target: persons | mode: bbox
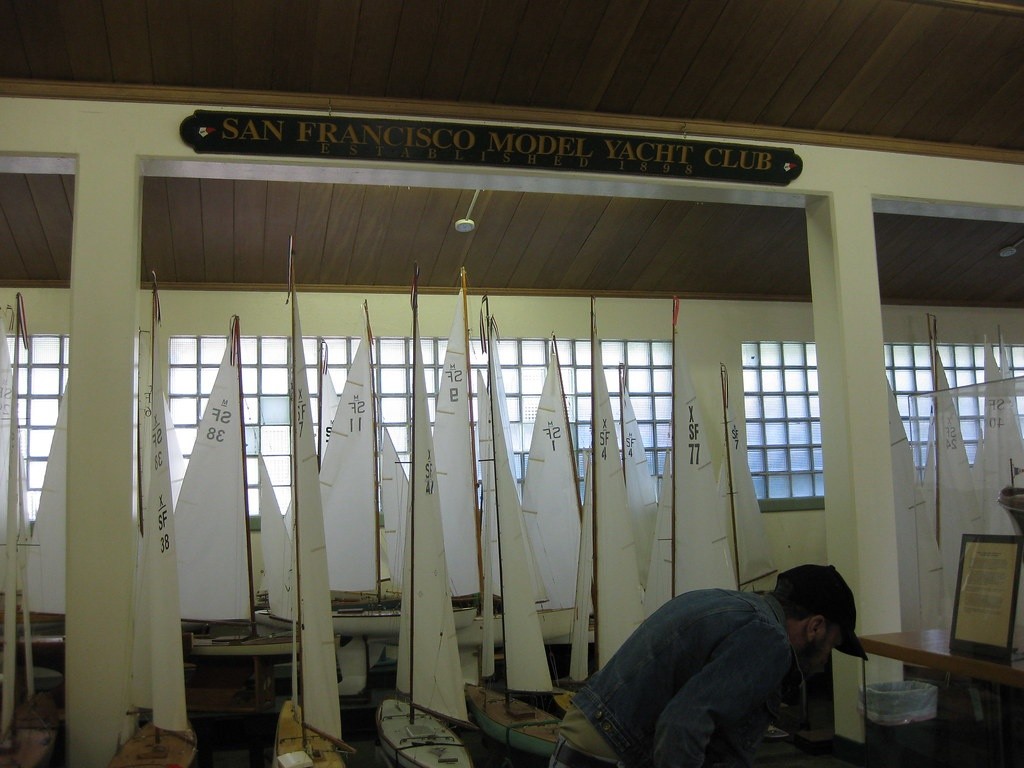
[547,565,869,767]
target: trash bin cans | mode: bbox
[859,680,938,768]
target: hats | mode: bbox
[774,565,868,661]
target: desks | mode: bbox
[862,627,1024,768]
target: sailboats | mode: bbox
[0,236,1024,768]
